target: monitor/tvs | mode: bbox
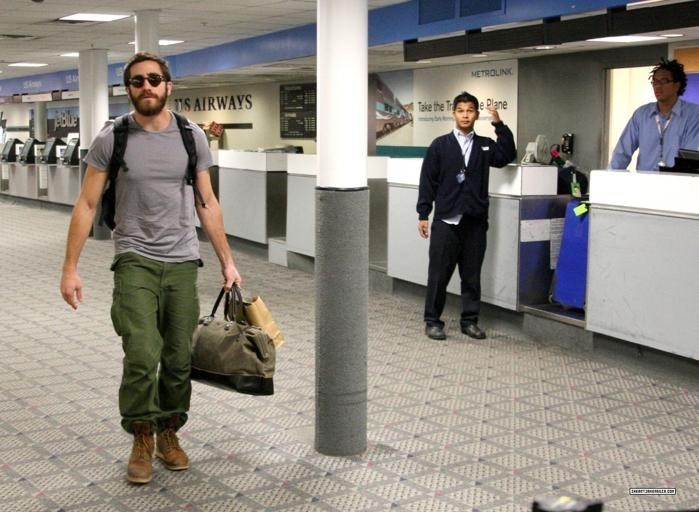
[20,138,38,161]
[40,137,57,162]
[2,138,25,161]
[64,138,79,162]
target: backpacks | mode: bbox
[101,111,199,231]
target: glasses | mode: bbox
[650,77,672,86]
[129,72,166,88]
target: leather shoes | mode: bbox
[461,323,486,339]
[425,324,446,340]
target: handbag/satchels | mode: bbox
[192,284,276,396]
[228,284,285,350]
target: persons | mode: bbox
[414,90,517,340]
[59,47,245,484]
[604,57,698,174]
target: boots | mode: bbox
[127,422,155,483]
[155,428,189,470]
